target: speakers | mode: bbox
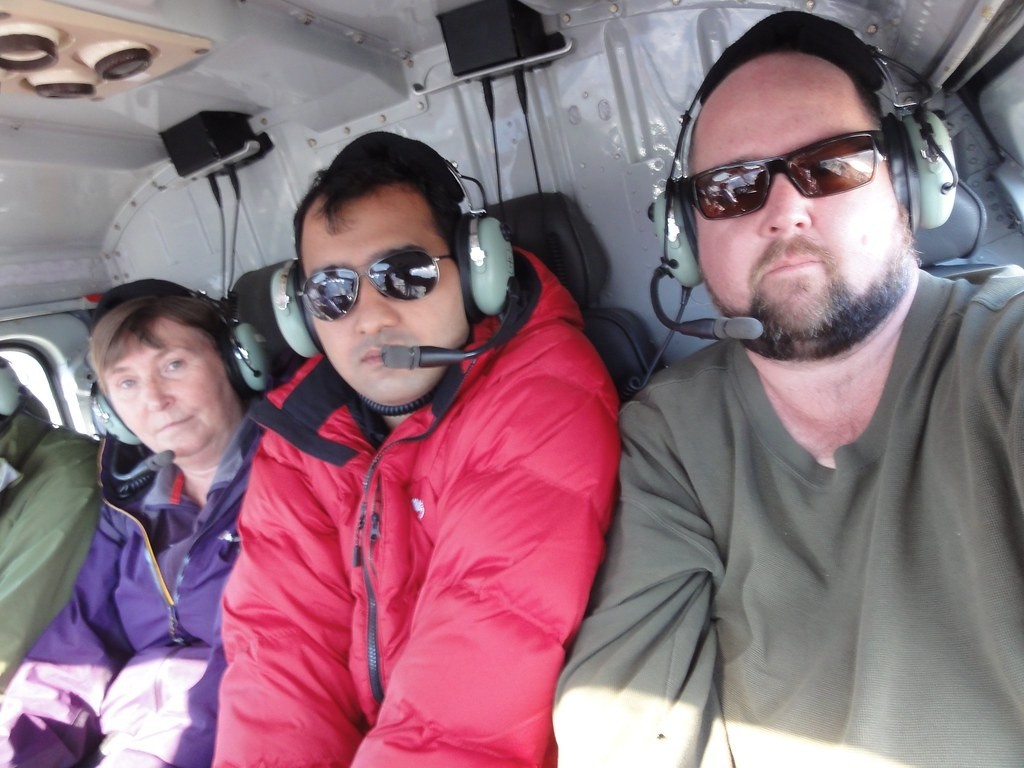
[437,0,547,77]
[158,110,257,176]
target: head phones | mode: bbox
[648,10,959,287]
[270,147,515,357]
[0,357,22,421]
[88,278,271,445]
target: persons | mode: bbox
[0,356,102,705]
[212,130,620,767]
[552,9,1023,766]
[1,278,266,768]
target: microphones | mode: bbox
[382,277,520,369]
[649,265,763,341]
[110,441,176,482]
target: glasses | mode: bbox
[298,251,455,321]
[681,129,889,220]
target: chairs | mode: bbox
[219,262,305,394]
[914,175,1007,286]
[473,189,658,414]
